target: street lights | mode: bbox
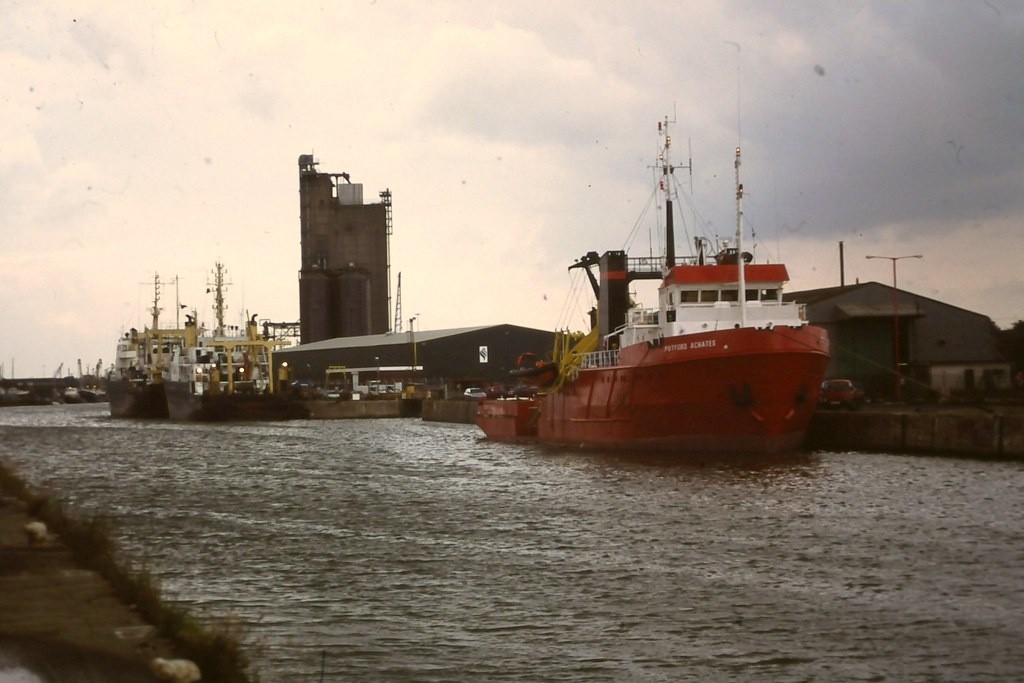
[865,254,923,399]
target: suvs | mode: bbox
[507,384,537,397]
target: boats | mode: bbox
[64,263,291,422]
[475,103,831,452]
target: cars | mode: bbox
[464,388,487,398]
[817,379,864,408]
[484,386,513,398]
